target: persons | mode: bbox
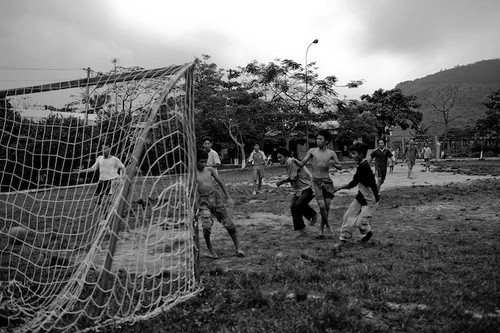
[74,144,126,224]
[368,140,395,197]
[420,142,432,173]
[292,130,342,238]
[202,138,221,175]
[247,143,270,196]
[402,138,422,179]
[388,147,396,172]
[189,152,245,257]
[334,142,379,248]
[275,148,318,235]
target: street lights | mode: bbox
[304,39,319,154]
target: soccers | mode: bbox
[9,227,27,245]
